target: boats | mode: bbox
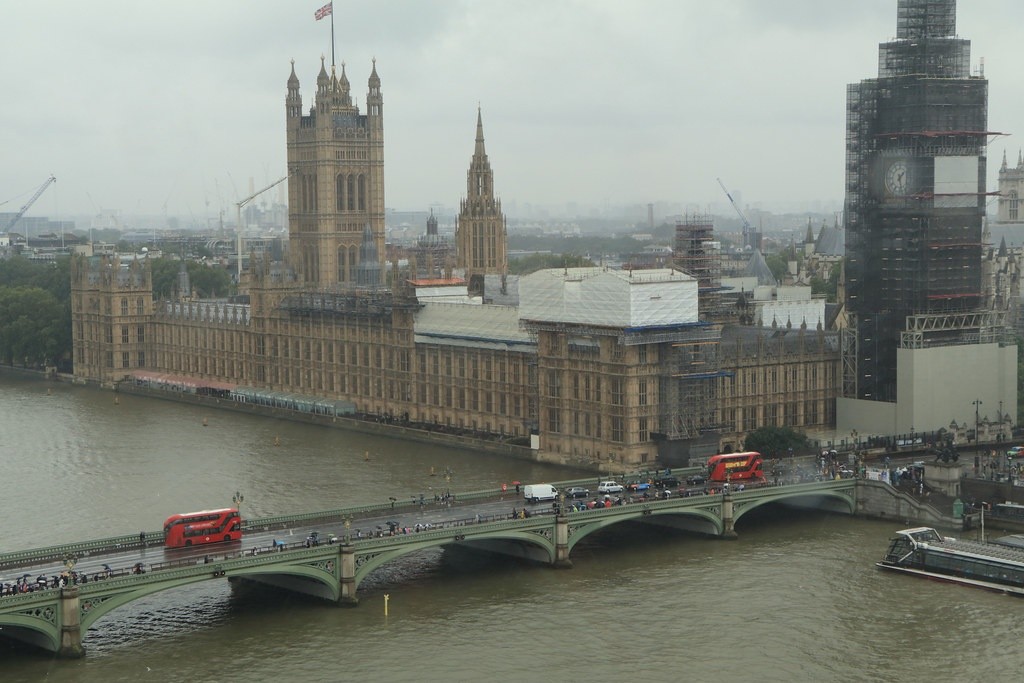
[875,526,1023,598]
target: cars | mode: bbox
[1006,446,1024,458]
[686,474,708,485]
[564,486,590,499]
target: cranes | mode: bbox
[0,175,58,238]
[236,172,295,287]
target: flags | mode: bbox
[313,2,334,21]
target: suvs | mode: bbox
[597,480,624,494]
[654,475,682,488]
[626,480,651,492]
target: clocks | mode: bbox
[885,160,915,196]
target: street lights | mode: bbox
[999,400,1003,456]
[724,467,734,496]
[62,550,79,588]
[971,397,982,454]
[232,490,245,511]
[556,486,567,517]
[910,425,914,463]
[341,513,354,545]
[981,501,988,542]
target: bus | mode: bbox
[162,508,244,548]
[706,451,764,481]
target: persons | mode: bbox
[0,529,154,598]
[202,466,745,564]
[758,450,936,506]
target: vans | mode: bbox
[524,483,559,502]
